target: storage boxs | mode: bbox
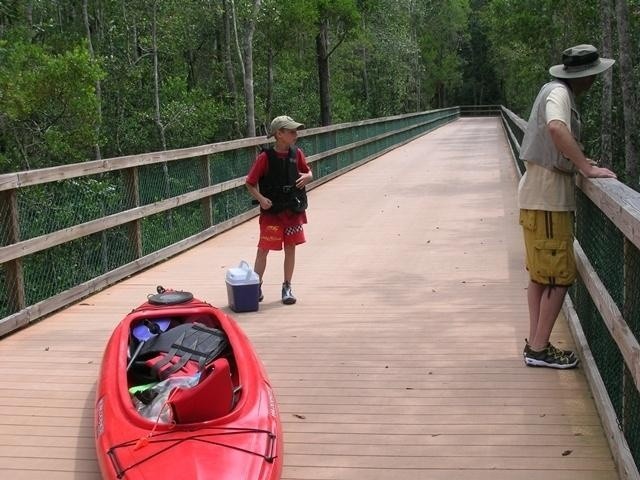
[223,259,263,312]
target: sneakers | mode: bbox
[522,337,579,369]
[281,282,296,304]
[257,281,264,301]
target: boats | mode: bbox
[92,286,284,480]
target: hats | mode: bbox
[266,116,305,139]
[548,44,616,79]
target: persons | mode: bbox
[244,115,314,305]
[517,43,619,370]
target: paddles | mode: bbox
[126,316,172,372]
